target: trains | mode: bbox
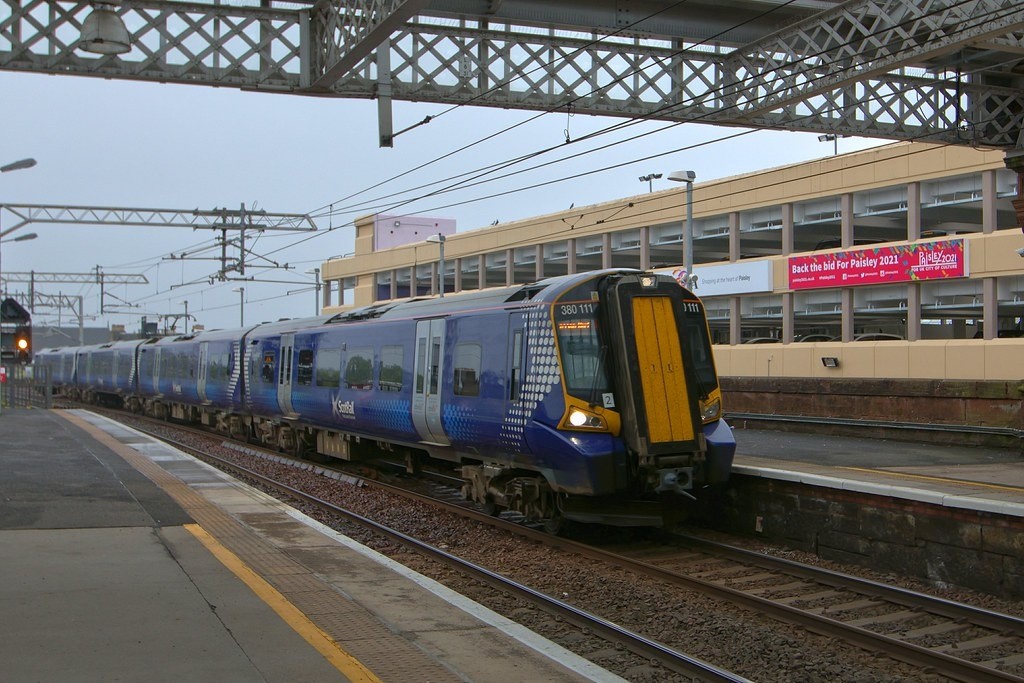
[34,268,735,535]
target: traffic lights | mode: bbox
[16,325,31,350]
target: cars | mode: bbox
[739,332,903,343]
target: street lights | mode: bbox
[426,233,446,298]
[667,170,695,295]
[0,233,38,246]
[233,288,244,326]
